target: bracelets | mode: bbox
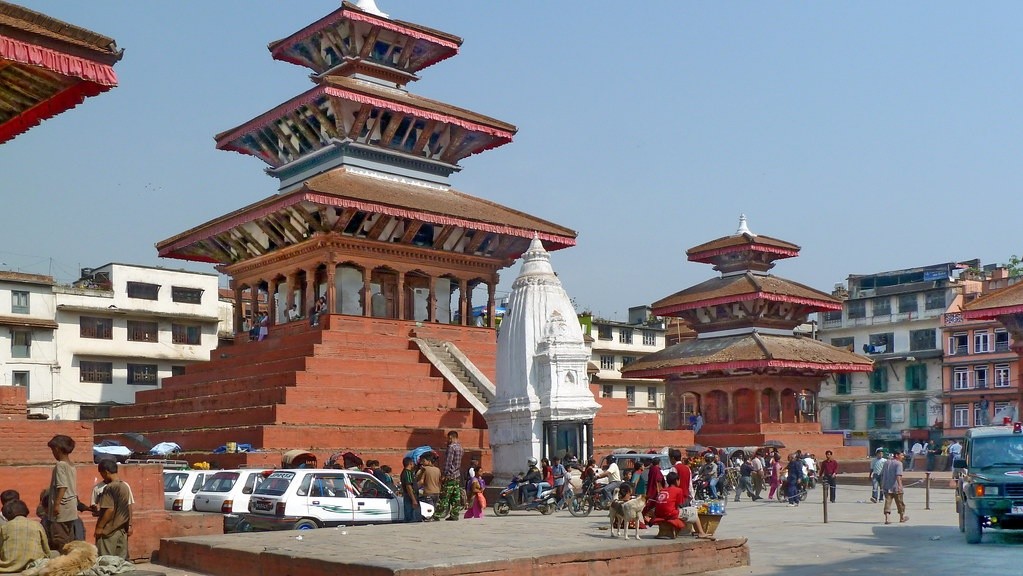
[129,525,132,526]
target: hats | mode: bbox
[774,452,781,459]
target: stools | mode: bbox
[654,522,681,540]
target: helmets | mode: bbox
[606,454,617,464]
[704,453,715,464]
[528,456,537,467]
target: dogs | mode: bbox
[607,494,650,540]
[20,540,98,576]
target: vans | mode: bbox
[598,452,675,490]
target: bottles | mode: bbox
[710,501,725,515]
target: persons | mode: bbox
[434,431,464,521]
[400,457,421,522]
[870,446,904,502]
[908,438,963,470]
[518,408,818,529]
[246,296,328,343]
[475,313,486,327]
[327,460,424,505]
[655,471,712,538]
[880,448,910,523]
[47,435,78,556]
[0,489,20,524]
[90,476,135,537]
[40,489,96,542]
[93,458,130,560]
[416,453,441,509]
[819,450,839,503]
[464,466,487,518]
[0,499,51,573]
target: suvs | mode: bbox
[955,417,1022,544]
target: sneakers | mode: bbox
[879,499,883,503]
[870,497,877,503]
[786,504,799,507]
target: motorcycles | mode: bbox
[692,466,727,502]
[494,472,558,516]
[568,469,636,516]
[776,469,808,502]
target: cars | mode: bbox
[163,470,220,512]
[245,469,435,531]
[192,468,275,533]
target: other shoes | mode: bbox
[533,498,540,503]
[445,516,459,521]
[426,516,440,522]
[901,516,909,522]
[884,521,891,524]
